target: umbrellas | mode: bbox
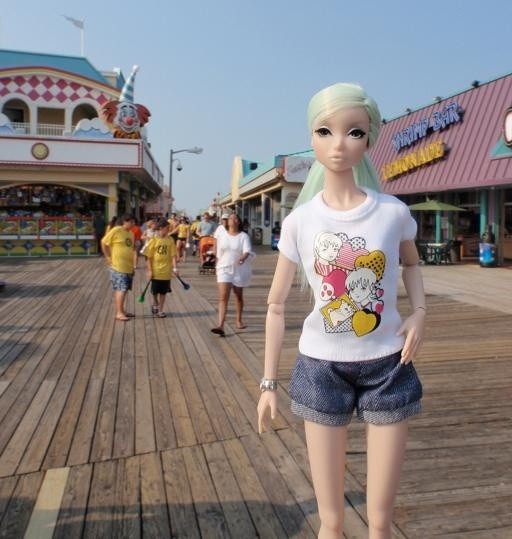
[407,199,467,262]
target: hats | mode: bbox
[221,214,229,219]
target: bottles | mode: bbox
[477,222,498,267]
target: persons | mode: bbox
[345,268,377,310]
[256,79,429,539]
[92,209,253,335]
[311,231,343,277]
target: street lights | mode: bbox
[169,147,202,217]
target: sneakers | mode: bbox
[192,251,195,256]
[211,327,225,336]
[237,322,245,329]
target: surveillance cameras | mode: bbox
[177,163,183,171]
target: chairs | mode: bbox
[415,240,462,265]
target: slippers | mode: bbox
[151,304,166,318]
[115,312,134,321]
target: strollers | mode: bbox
[198,236,218,275]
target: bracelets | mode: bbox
[259,378,278,392]
[413,307,426,312]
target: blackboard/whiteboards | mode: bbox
[460,237,480,260]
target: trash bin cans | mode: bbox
[252,227,262,245]
